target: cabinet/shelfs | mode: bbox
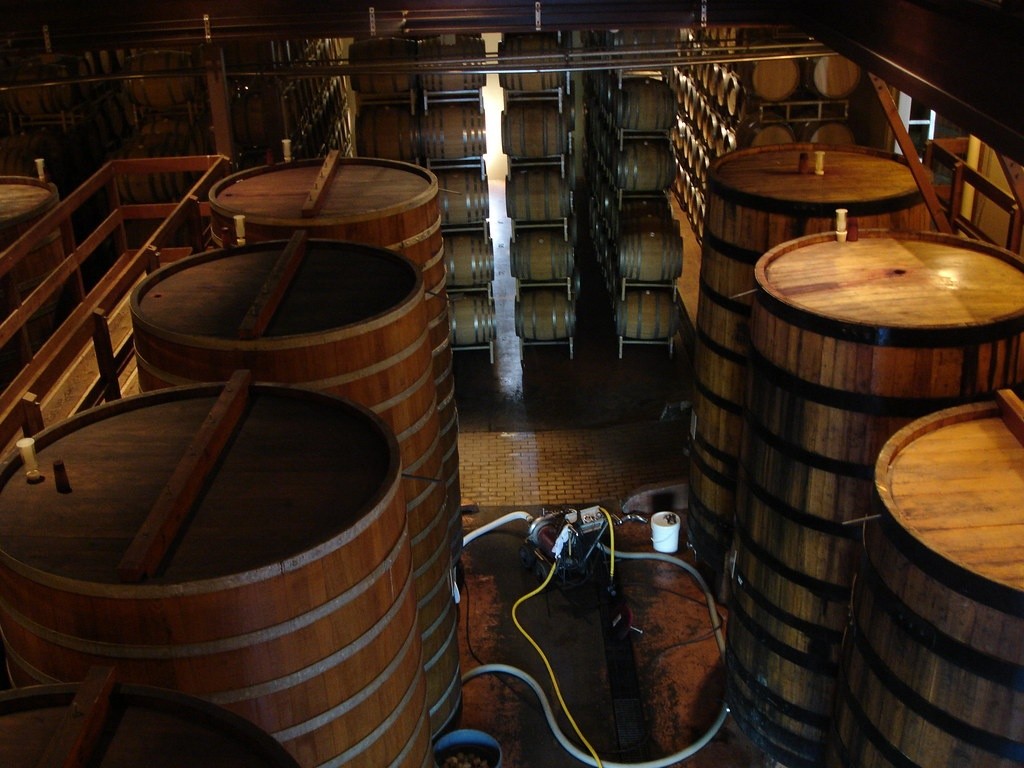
[0,29,1024,365]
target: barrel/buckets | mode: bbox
[129,230,464,743]
[689,141,936,573]
[0,175,77,395]
[431,729,503,768]
[724,225,1024,768]
[650,511,681,553]
[824,388,1024,768]
[207,148,464,569]
[0,367,435,768]
[0,663,301,768]
